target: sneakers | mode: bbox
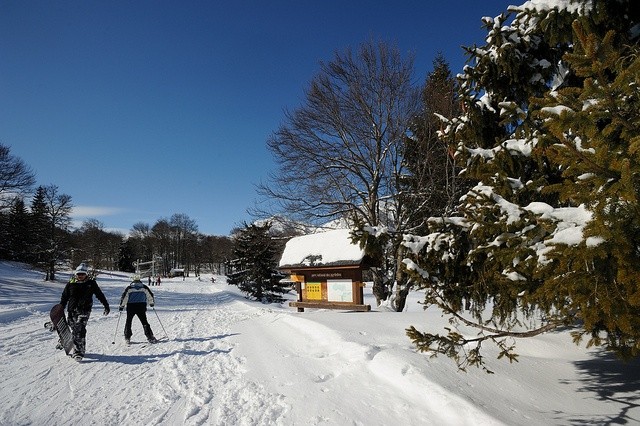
[72,352,85,362]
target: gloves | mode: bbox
[103,305,110,315]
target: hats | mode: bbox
[75,262,88,276]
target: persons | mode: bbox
[152,277,155,286]
[155,277,158,285]
[148,276,151,286]
[60,263,110,362]
[119,273,157,344]
[157,276,160,286]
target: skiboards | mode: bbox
[126,337,165,349]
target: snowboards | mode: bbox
[44,304,74,355]
